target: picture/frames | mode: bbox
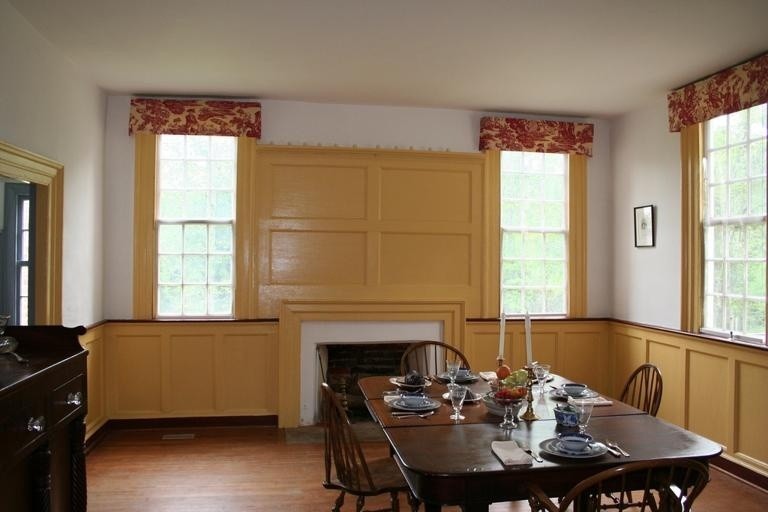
[633,204,655,247]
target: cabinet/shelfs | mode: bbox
[1,323,93,510]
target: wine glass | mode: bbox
[534,365,550,399]
[573,399,593,435]
[449,388,466,420]
[442,359,460,387]
[492,397,522,430]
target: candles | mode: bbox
[497,312,535,367]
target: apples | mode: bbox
[496,364,528,398]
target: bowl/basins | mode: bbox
[482,395,522,417]
[560,432,591,451]
[562,383,586,395]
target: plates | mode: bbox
[552,387,598,400]
[531,375,553,384]
[540,438,606,459]
[388,375,482,411]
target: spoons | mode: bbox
[609,441,630,456]
[397,412,434,419]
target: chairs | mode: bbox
[526,364,710,512]
[316,338,470,512]
